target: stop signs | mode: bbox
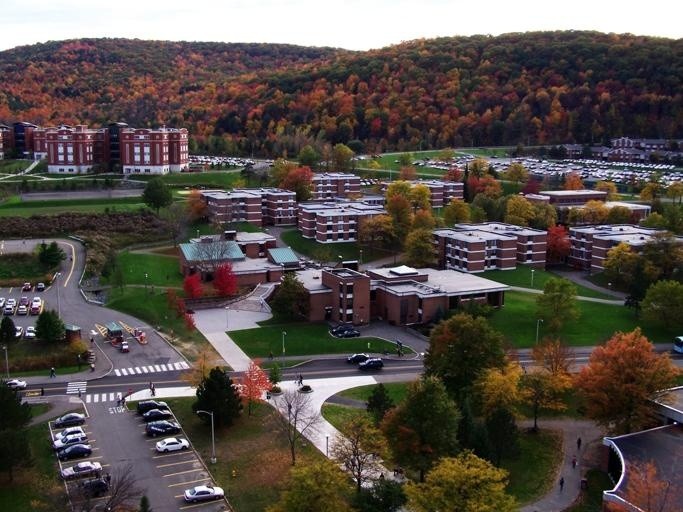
[127,388,132,394]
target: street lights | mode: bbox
[2,346,10,378]
[281,330,286,353]
[196,228,200,238]
[359,249,362,264]
[224,306,229,331]
[535,318,543,344]
[53,271,61,323]
[195,408,216,463]
[142,272,148,292]
[530,269,535,288]
[606,282,611,299]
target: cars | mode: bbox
[183,484,225,502]
[21,282,30,291]
[50,411,109,500]
[360,177,381,186]
[0,296,41,316]
[136,398,189,453]
[35,282,44,290]
[14,325,23,338]
[393,159,401,163]
[347,352,368,363]
[4,378,27,390]
[331,324,353,334]
[338,329,360,338]
[265,159,275,168]
[411,153,682,191]
[187,155,256,168]
[24,326,35,338]
[318,152,384,166]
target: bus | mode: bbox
[673,336,683,354]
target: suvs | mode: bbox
[358,357,385,370]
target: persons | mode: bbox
[114,395,120,407]
[285,400,292,415]
[297,372,304,387]
[205,479,211,486]
[118,395,125,409]
[76,354,83,371]
[379,471,384,477]
[383,346,392,360]
[558,475,564,493]
[570,454,577,468]
[105,472,111,487]
[148,380,153,392]
[149,383,156,397]
[293,370,299,383]
[39,386,44,396]
[395,340,404,357]
[48,367,56,378]
[267,350,274,361]
[576,436,581,450]
[93,468,102,478]
[90,336,94,348]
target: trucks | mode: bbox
[105,322,127,352]
